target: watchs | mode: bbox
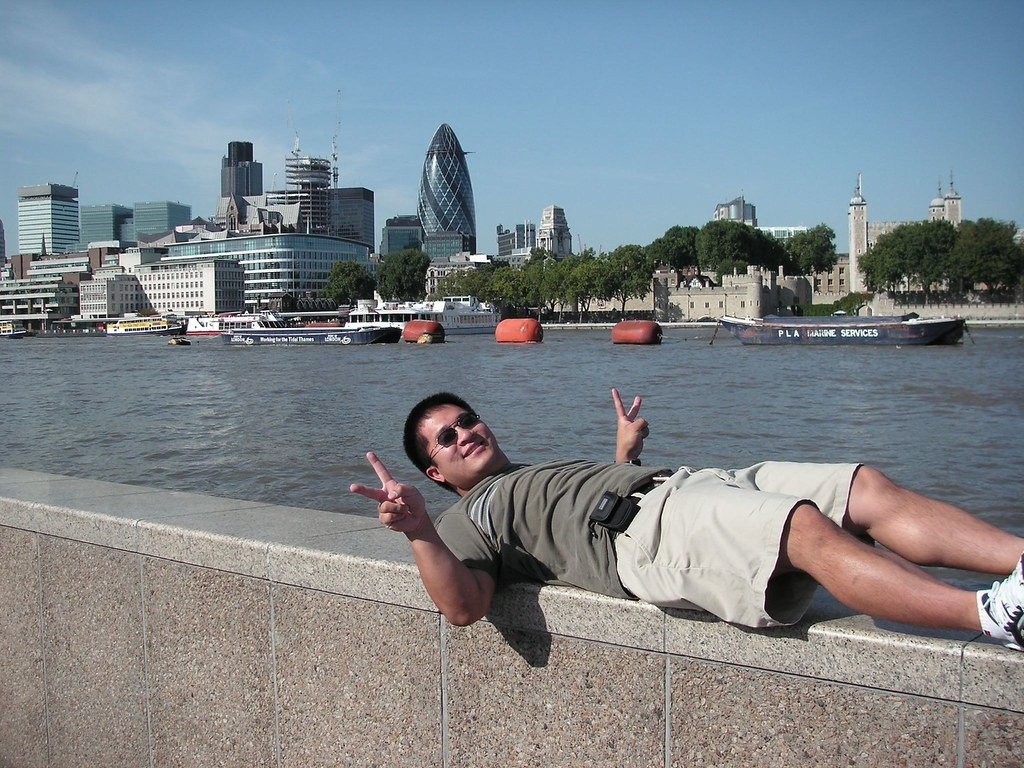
[626,459,642,467]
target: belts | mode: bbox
[608,471,675,544]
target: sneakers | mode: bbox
[976,550,1024,652]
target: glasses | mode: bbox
[428,413,480,468]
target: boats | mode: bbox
[105,312,182,332]
[719,314,965,345]
[344,290,502,335]
[221,327,403,345]
[0,319,26,339]
[186,309,283,336]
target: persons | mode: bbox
[348,387,1024,656]
